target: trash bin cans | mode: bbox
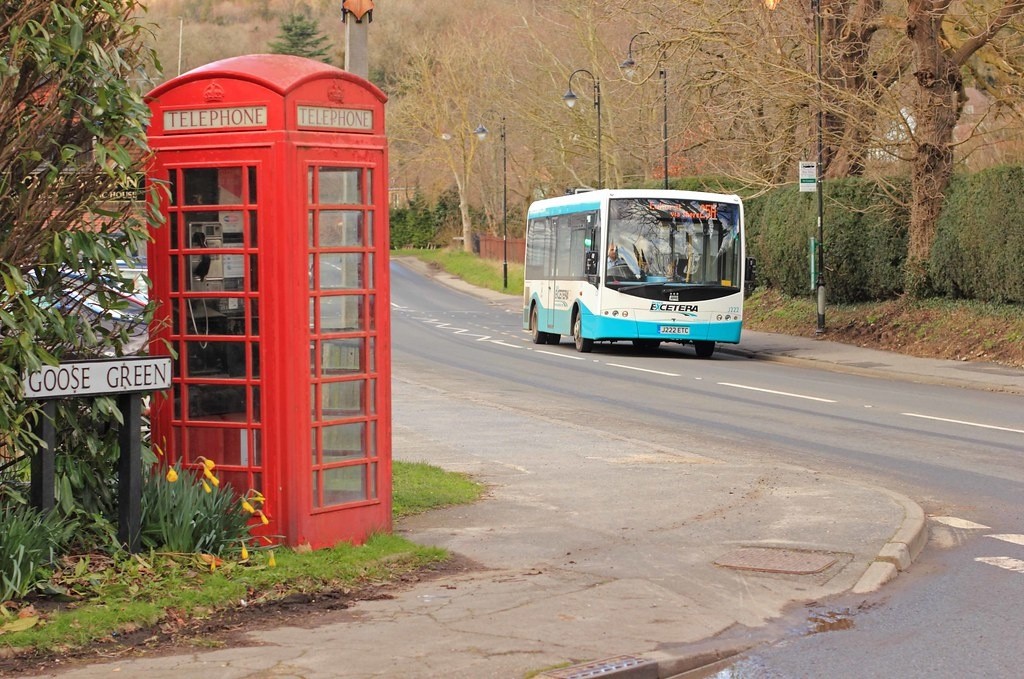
[473,236,480,252]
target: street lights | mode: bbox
[617,30,670,189]
[474,110,510,290]
[765,0,828,336]
[564,69,602,188]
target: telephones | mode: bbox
[188,220,225,300]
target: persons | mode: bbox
[607,244,627,268]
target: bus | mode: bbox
[522,188,758,359]
